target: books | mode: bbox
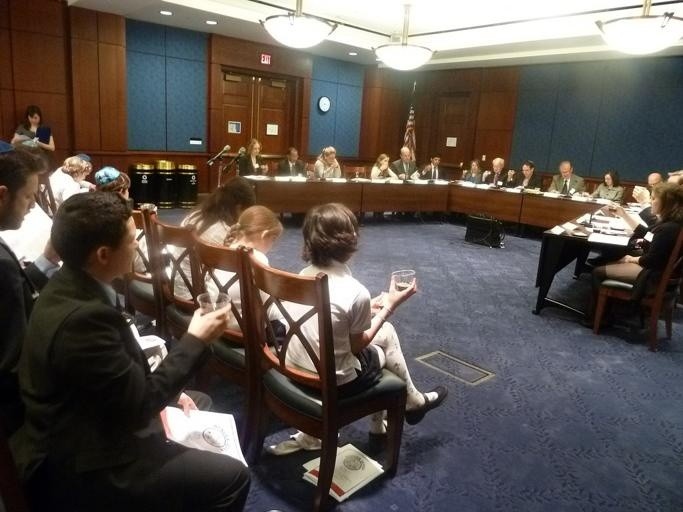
[302,443,383,500]
[159,405,247,466]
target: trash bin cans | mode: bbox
[175,164,199,209]
[130,162,156,210]
[155,160,176,209]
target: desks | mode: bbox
[450,171,523,226]
[532,208,643,315]
[520,175,612,228]
[362,163,450,222]
[241,160,362,220]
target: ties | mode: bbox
[433,166,437,179]
[404,162,410,172]
[495,173,498,184]
[291,163,295,174]
[561,178,568,194]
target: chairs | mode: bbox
[243,245,408,504]
[592,232,681,352]
[124,204,155,327]
[148,208,195,345]
[190,225,244,412]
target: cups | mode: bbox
[390,268,416,293]
[140,202,155,212]
[607,202,618,215]
[196,292,230,323]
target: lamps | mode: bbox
[594,0,683,58]
[258,0,340,50]
[371,4,438,72]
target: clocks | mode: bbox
[318,96,331,113]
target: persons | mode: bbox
[578,182,682,329]
[459,158,624,206]
[8,190,249,511]
[10,104,56,156]
[204,203,286,339]
[0,139,174,279]
[0,140,62,461]
[164,176,258,317]
[236,138,447,226]
[278,202,448,458]
[609,169,682,265]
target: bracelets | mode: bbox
[386,308,393,317]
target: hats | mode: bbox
[94,166,122,186]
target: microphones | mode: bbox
[222,147,245,174]
[206,144,231,167]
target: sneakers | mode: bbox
[406,386,448,426]
[368,416,392,453]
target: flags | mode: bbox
[402,92,417,151]
